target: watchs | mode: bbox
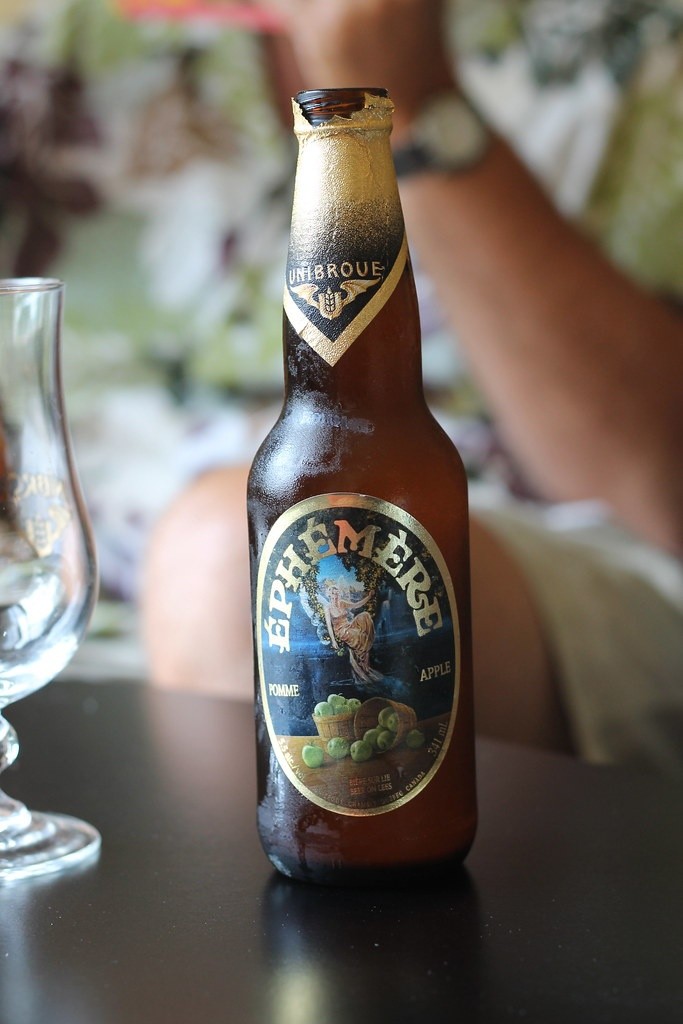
[389,94,490,172]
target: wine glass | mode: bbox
[0,279,101,882]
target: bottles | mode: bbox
[246,88,474,887]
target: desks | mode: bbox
[0,677,682,1024]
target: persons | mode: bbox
[145,1,683,748]
